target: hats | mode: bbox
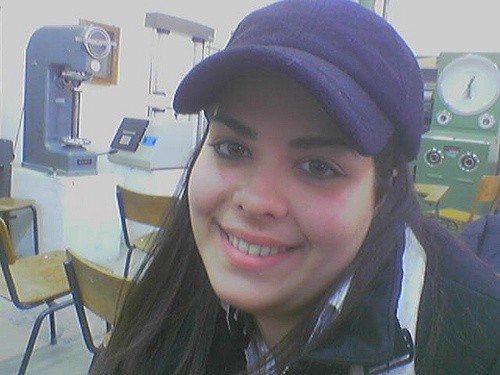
[172,0,425,159]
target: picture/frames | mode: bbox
[76,19,121,86]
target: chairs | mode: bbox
[0,196,40,255]
[114,185,180,279]
[427,174,500,237]
[63,245,142,354]
[0,217,112,375]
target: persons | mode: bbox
[87,0,500,375]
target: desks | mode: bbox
[7,162,190,269]
[415,183,450,221]
